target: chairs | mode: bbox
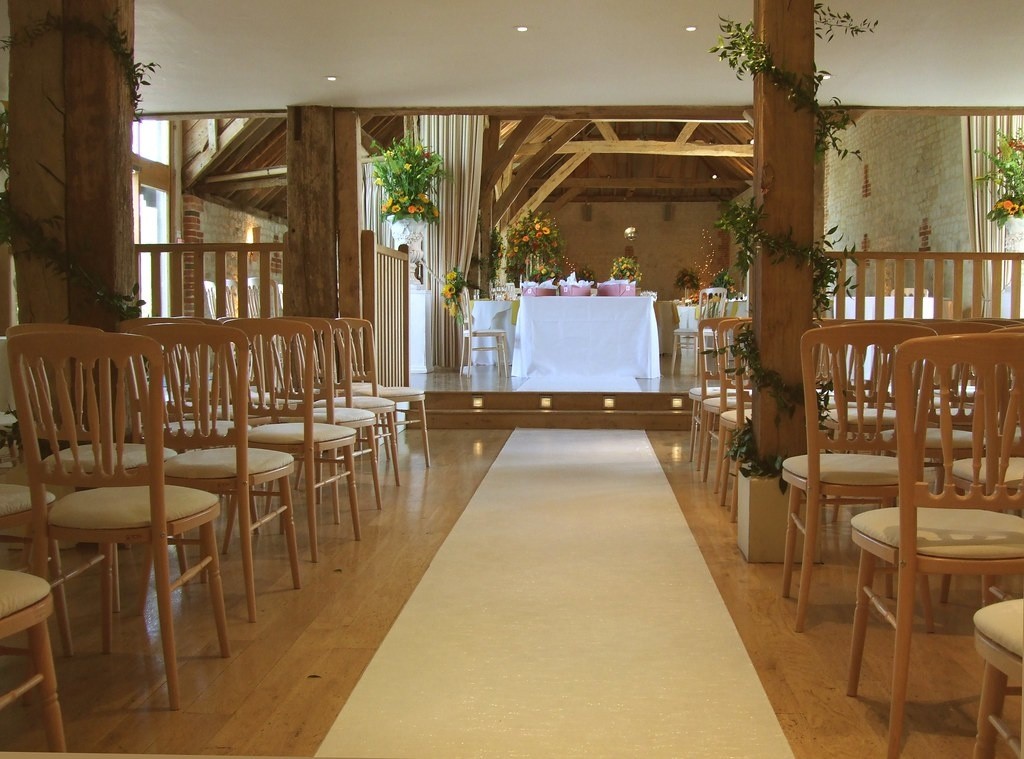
[460,286,509,379]
[670,288,728,377]
[688,317,1024,759]
[203,277,282,322]
[0,315,430,752]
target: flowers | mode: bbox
[370,130,455,225]
[491,226,506,284]
[609,256,642,285]
[441,267,467,325]
[972,128,1024,230]
[505,209,568,284]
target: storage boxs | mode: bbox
[559,285,591,297]
[597,284,636,297]
[523,287,556,296]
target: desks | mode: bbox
[511,295,660,378]
[827,297,934,386]
[469,300,520,366]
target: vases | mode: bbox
[386,215,429,289]
